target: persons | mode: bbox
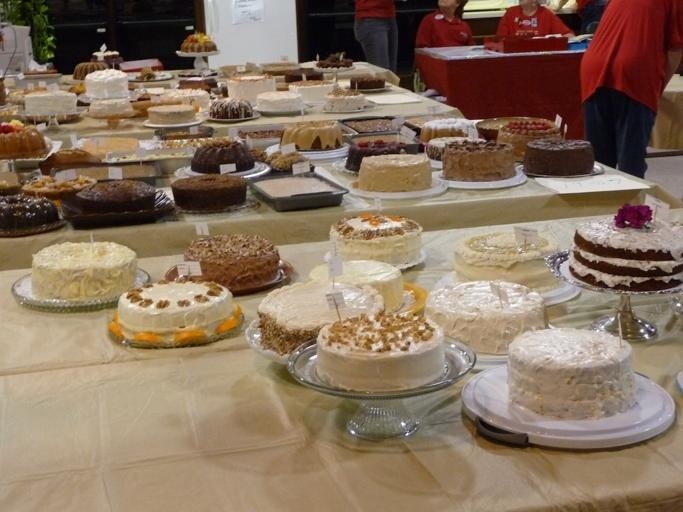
[415,0,476,48]
[353,0,398,76]
[580,0,683,179]
[496,0,574,41]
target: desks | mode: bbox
[416,34,589,142]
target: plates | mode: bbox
[80,110,140,130]
[435,268,583,308]
[467,348,516,366]
[9,267,152,314]
[78,91,139,105]
[288,142,350,161]
[322,100,377,114]
[284,335,476,442]
[475,116,541,140]
[542,248,681,343]
[366,92,422,105]
[59,190,175,230]
[348,177,447,199]
[128,73,175,83]
[169,197,262,214]
[21,73,62,79]
[207,112,264,123]
[431,167,528,189]
[458,362,677,452]
[345,132,420,157]
[394,279,429,318]
[176,49,219,73]
[161,258,294,295]
[330,158,360,175]
[254,104,313,115]
[516,162,605,179]
[103,311,244,350]
[0,140,63,169]
[173,160,272,182]
[244,316,294,369]
[16,109,81,132]
[324,250,429,272]
[341,115,420,134]
[346,85,392,93]
[0,219,69,237]
[141,118,205,131]
[247,170,350,212]
[312,66,356,73]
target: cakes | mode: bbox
[325,87,364,111]
[507,328,636,419]
[22,175,92,195]
[309,260,406,314]
[497,118,562,162]
[407,114,455,127]
[257,278,385,354]
[184,233,280,290]
[253,175,342,197]
[345,139,409,172]
[524,139,594,177]
[109,280,245,349]
[420,118,483,142]
[0,105,19,117]
[249,149,268,161]
[237,129,284,140]
[285,68,323,82]
[24,91,78,115]
[454,230,563,294]
[477,117,532,145]
[171,174,247,210]
[316,53,355,72]
[289,79,337,101]
[1,119,51,160]
[111,147,190,163]
[77,179,156,213]
[72,84,85,95]
[78,137,138,157]
[162,137,227,150]
[0,170,22,189]
[348,154,432,193]
[191,142,254,174]
[92,43,123,70]
[179,78,217,91]
[74,61,109,80]
[136,66,155,82]
[315,314,445,393]
[181,33,216,53]
[220,61,260,81]
[226,75,276,105]
[161,88,210,111]
[129,89,158,115]
[165,130,205,138]
[254,91,303,112]
[280,121,343,151]
[211,82,226,96]
[568,204,683,290]
[425,280,548,356]
[352,74,384,89]
[265,150,306,171]
[263,61,300,75]
[342,118,402,134]
[147,103,197,124]
[53,149,99,167]
[85,69,129,99]
[350,133,414,144]
[443,140,516,182]
[329,214,422,266]
[425,136,487,162]
[209,96,253,120]
[32,241,138,298]
[0,194,59,228]
[7,88,45,103]
[88,98,134,115]
